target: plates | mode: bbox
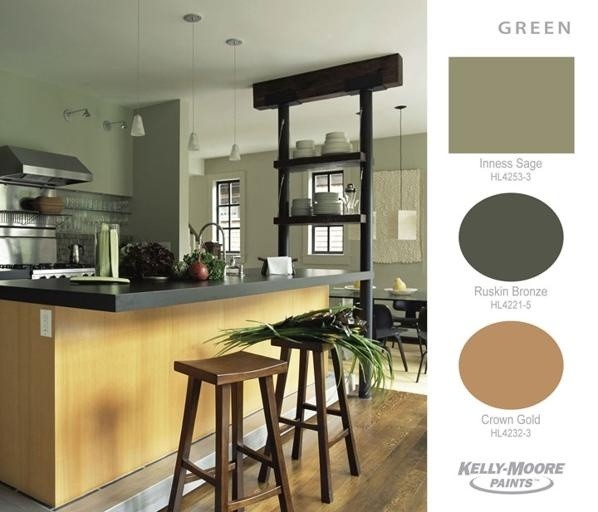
[345,285,377,293]
[312,192,343,216]
[291,199,311,217]
[384,288,418,296]
[293,140,316,158]
[321,132,352,156]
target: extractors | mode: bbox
[0,144,93,189]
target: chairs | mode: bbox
[414,309,428,382]
[392,299,424,358]
[357,306,410,374]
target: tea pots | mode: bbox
[67,244,84,263]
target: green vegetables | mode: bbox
[203,303,395,404]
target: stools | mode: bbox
[270,327,360,505]
[167,351,295,511]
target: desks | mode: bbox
[3,268,374,510]
[329,286,427,303]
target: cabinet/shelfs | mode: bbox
[1,208,74,230]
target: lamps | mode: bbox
[129,2,146,137]
[102,120,129,135]
[387,103,417,243]
[183,14,203,150]
[225,38,243,160]
[62,108,91,120]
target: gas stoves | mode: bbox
[2,262,95,280]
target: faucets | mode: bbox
[196,222,245,277]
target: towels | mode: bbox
[265,255,293,276]
